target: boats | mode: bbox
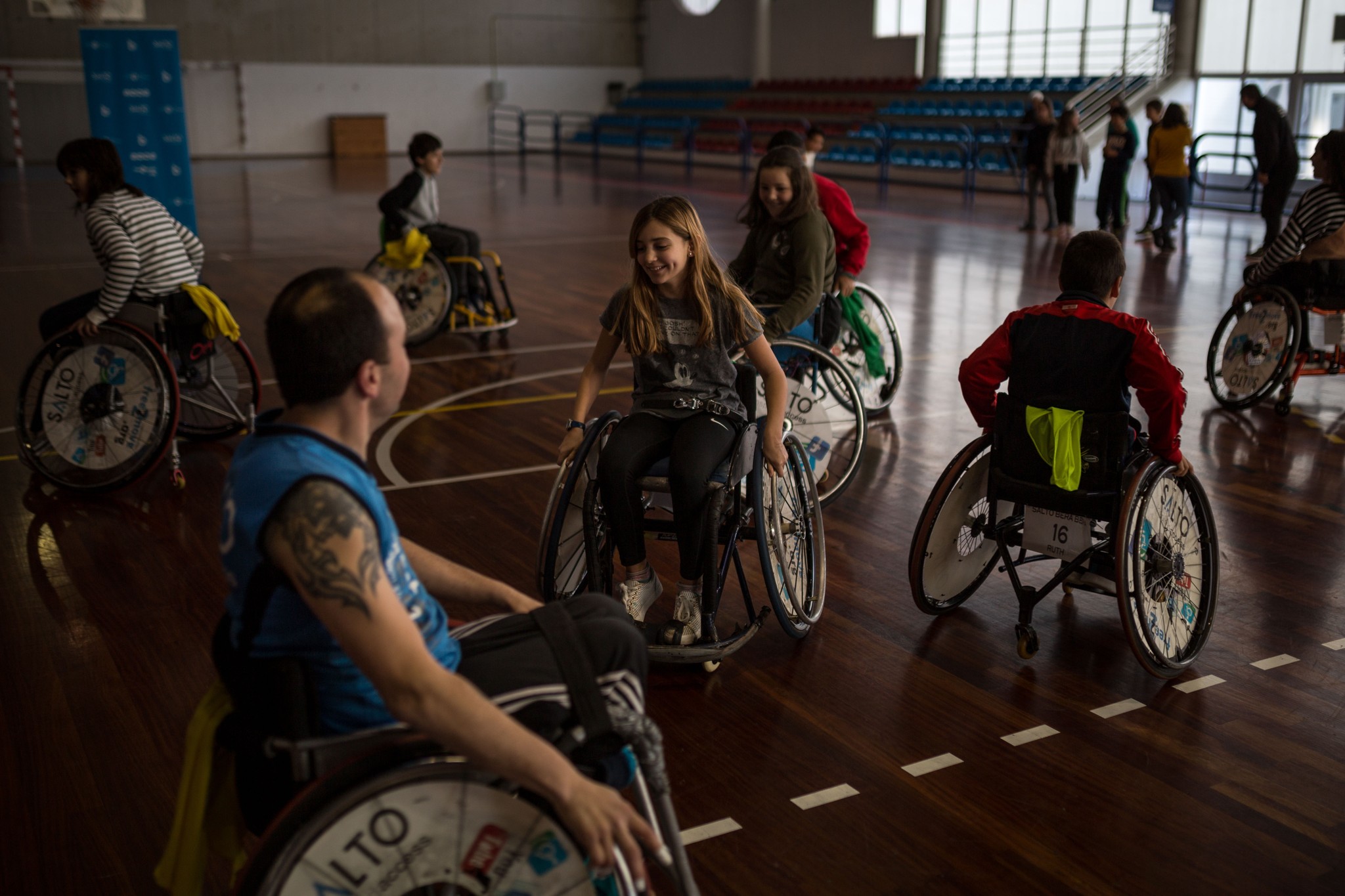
[366,239,518,349]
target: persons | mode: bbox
[1241,129,1345,358]
[377,131,488,316]
[38,137,205,358]
[561,193,788,643]
[1047,105,1092,231]
[762,127,868,362]
[958,230,1199,526]
[1241,84,1301,258]
[800,129,827,171]
[1007,88,1059,231]
[223,269,676,893]
[1096,100,1196,253]
[696,142,839,390]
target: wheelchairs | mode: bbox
[908,392,1220,679]
[1205,282,1345,417]
[534,280,903,667]
[214,705,699,896]
[15,285,262,493]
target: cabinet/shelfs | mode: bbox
[331,116,386,160]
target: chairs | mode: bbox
[566,76,1149,176]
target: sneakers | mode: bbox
[665,590,701,645]
[617,564,663,622]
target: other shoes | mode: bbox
[1055,559,1084,585]
[1080,550,1116,593]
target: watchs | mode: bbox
[565,418,587,432]
